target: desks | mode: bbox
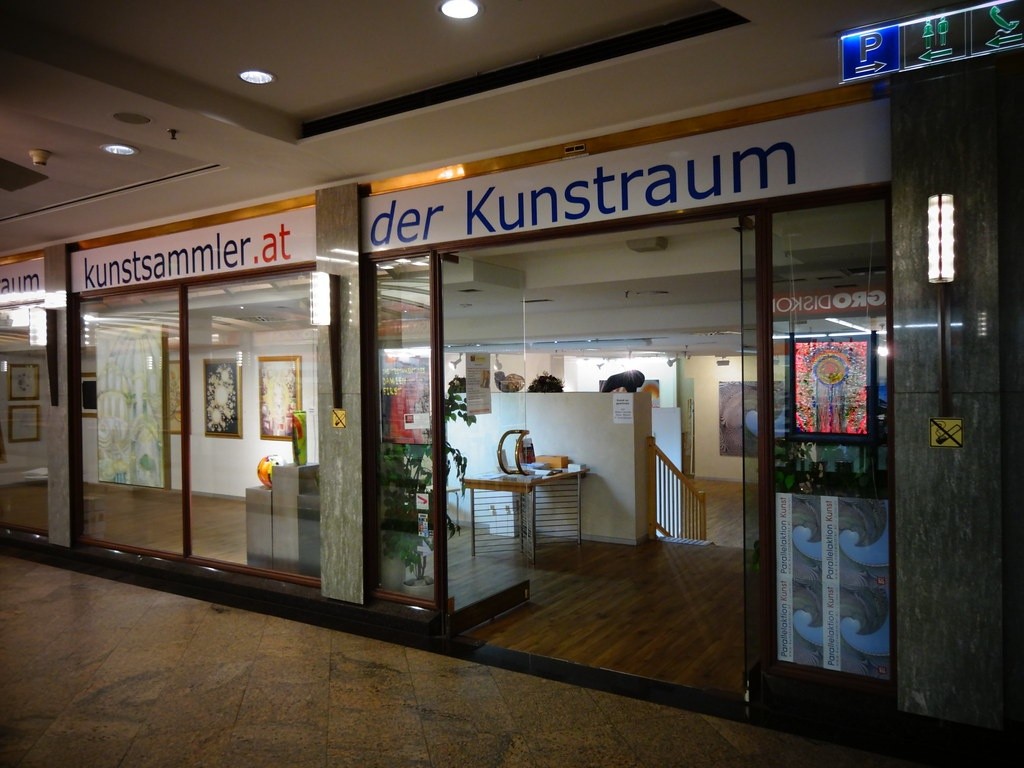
[461,464,588,566]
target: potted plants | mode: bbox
[773,437,827,495]
[376,356,476,588]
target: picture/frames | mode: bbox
[81,372,97,418]
[168,360,192,434]
[8,405,40,444]
[95,324,172,490]
[257,356,301,441]
[7,363,40,400]
[203,358,243,440]
[784,330,877,443]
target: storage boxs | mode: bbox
[535,454,569,468]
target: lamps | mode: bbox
[666,353,680,368]
[29,306,59,406]
[927,193,957,417]
[494,354,503,371]
[449,353,463,370]
[309,270,343,409]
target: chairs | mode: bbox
[403,454,460,525]
